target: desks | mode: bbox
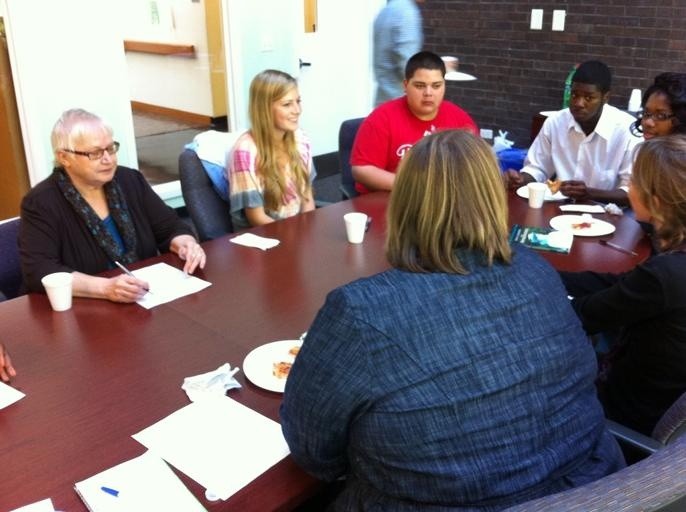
[0,173,651,512]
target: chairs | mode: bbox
[338,115,371,197]
[496,421,686,512]
[175,133,236,237]
[0,214,36,300]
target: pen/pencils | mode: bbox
[115,261,155,295]
[599,239,639,257]
[101,487,139,506]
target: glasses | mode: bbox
[634,111,681,122]
[60,142,121,161]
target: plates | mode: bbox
[516,184,570,200]
[243,340,304,393]
[549,215,616,236]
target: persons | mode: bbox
[17,108,208,303]
[0,341,17,384]
[348,51,480,194]
[503,59,645,206]
[596,135,686,434]
[278,128,628,512]
[371,0,426,109]
[226,69,318,231]
[629,71,686,141]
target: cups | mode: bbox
[41,272,73,312]
[527,182,547,209]
[344,212,368,244]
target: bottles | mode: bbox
[563,65,579,108]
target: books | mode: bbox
[508,223,575,255]
